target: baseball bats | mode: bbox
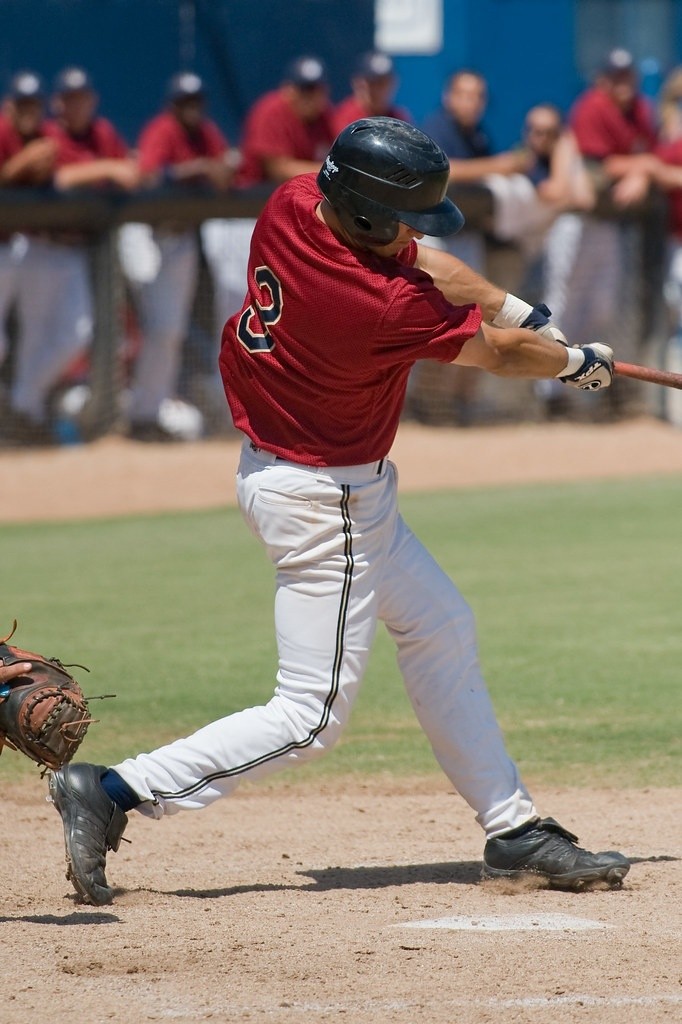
[613,361,682,390]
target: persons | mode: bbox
[46,121,632,905]
[0,633,31,689]
[0,47,682,435]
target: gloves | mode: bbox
[555,340,613,392]
[492,294,569,345]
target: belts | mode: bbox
[243,437,389,480]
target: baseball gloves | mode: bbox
[0,638,91,772]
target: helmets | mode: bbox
[316,115,465,246]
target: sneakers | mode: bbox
[48,762,128,908]
[481,817,630,892]
